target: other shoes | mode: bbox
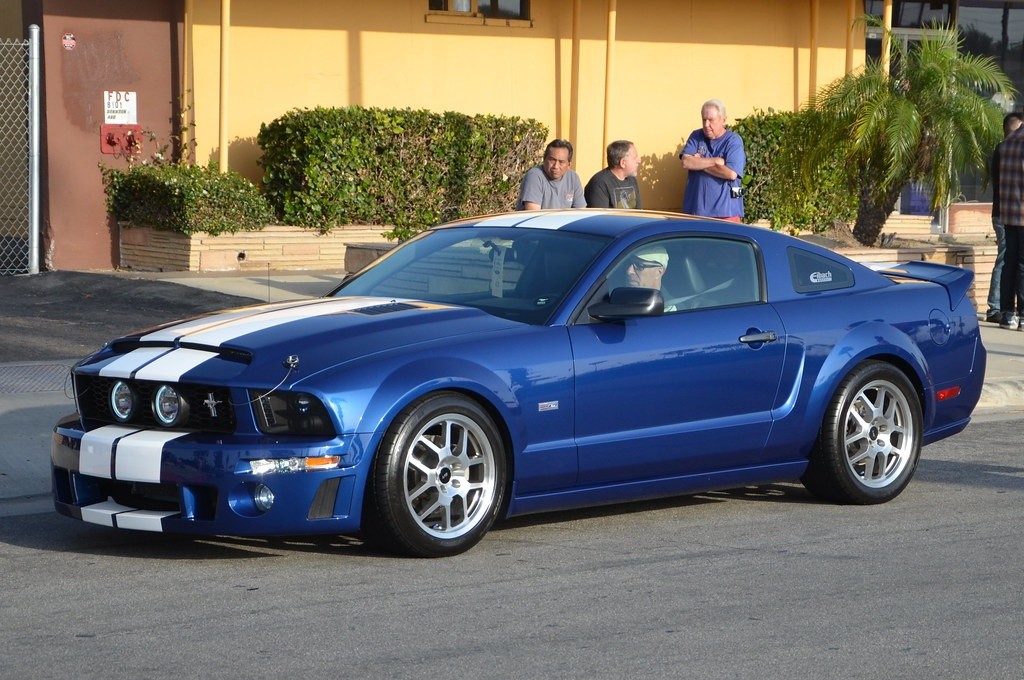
[987,310,1002,323]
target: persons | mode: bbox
[584,141,641,209]
[624,244,679,312]
[986,111,1024,324]
[998,123,1024,331]
[516,139,588,211]
[679,99,746,221]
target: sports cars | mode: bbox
[50,206,989,561]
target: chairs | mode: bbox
[665,252,720,309]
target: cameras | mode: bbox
[731,187,745,198]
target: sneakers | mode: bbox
[999,312,1024,332]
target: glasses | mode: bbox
[630,257,662,270]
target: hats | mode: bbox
[631,244,669,271]
